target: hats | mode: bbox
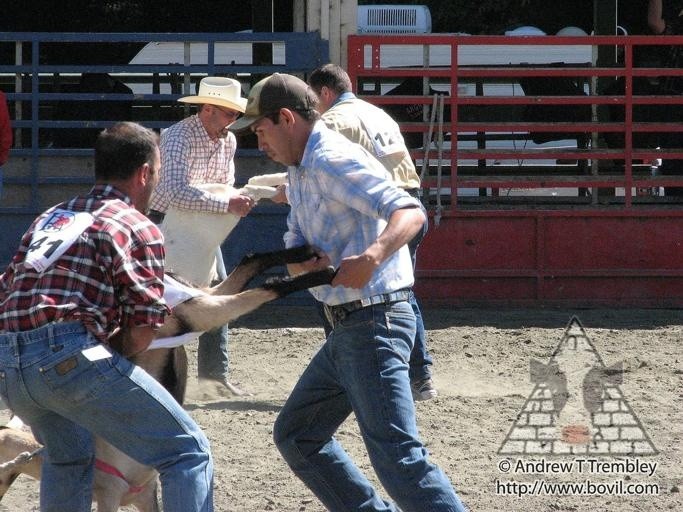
[178,76,248,113]
[225,72,316,130]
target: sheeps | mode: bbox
[0,244,336,512]
[159,172,292,289]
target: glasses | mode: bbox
[220,109,240,119]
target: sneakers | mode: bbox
[200,379,248,396]
[410,377,437,399]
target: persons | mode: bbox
[220,69,467,512]
[645,0,682,199]
[140,76,259,398]
[269,61,437,404]
[0,121,216,512]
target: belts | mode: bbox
[322,292,408,328]
[405,188,419,196]
